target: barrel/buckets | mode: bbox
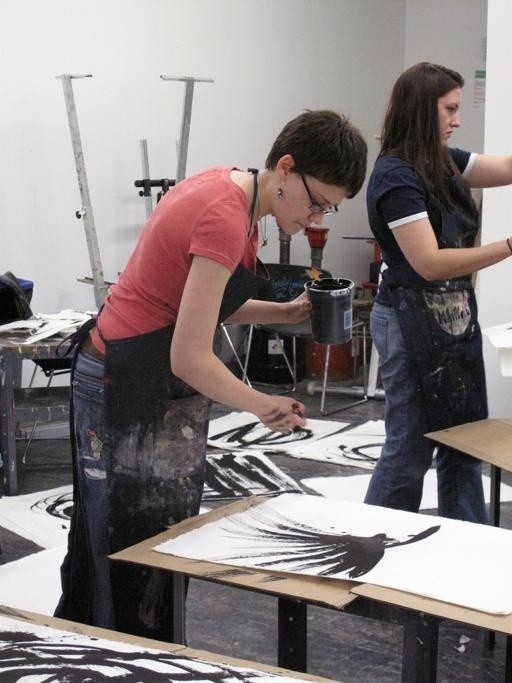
[304,278,355,345]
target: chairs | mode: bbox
[0,270,84,467]
[239,256,372,414]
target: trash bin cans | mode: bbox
[241,264,332,383]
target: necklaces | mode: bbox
[257,186,269,248]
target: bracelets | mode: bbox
[507,239,512,252]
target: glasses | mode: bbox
[301,173,339,217]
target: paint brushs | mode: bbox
[291,402,303,433]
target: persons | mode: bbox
[52,110,368,647]
[364,61,512,525]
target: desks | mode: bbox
[1,323,80,487]
[2,415,512,683]
